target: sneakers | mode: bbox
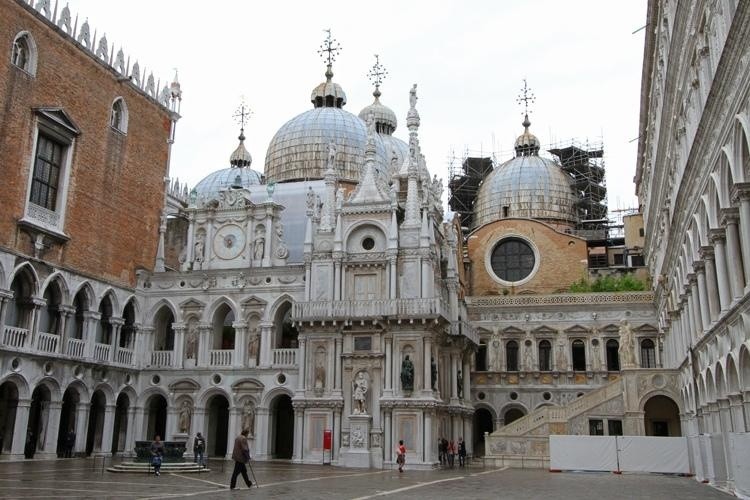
[231,487,240,491]
[249,482,256,490]
[156,471,160,477]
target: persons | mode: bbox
[178,401,190,433]
[241,399,254,440]
[431,356,437,390]
[248,328,258,357]
[184,327,196,358]
[456,370,462,398]
[229,427,256,493]
[395,440,406,474]
[400,354,415,389]
[194,431,207,467]
[353,372,369,414]
[150,435,166,478]
[617,318,635,364]
[437,436,466,469]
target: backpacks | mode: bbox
[151,456,161,466]
